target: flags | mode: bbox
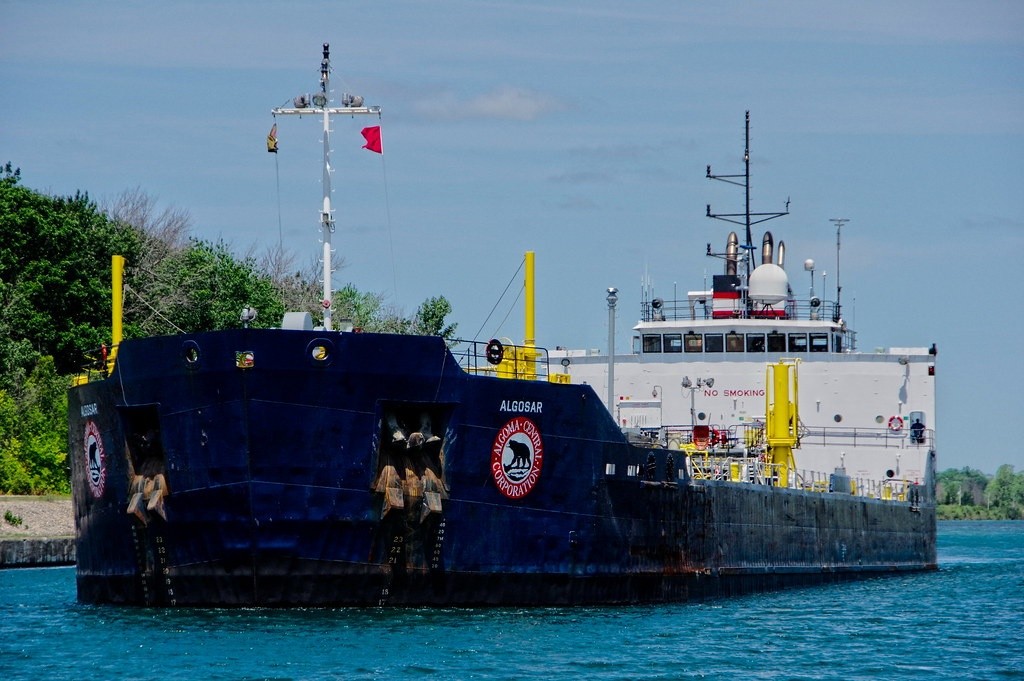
[267,124,278,154]
[360,125,383,155]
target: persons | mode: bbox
[911,418,925,438]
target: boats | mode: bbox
[66,42,940,608]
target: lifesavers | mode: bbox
[707,429,719,446]
[888,416,903,431]
[486,339,504,365]
[721,430,726,444]
[323,300,331,308]
